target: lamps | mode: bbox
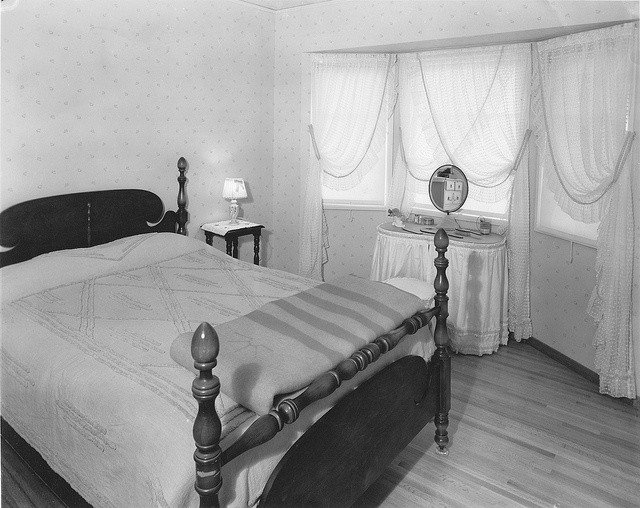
[222,177,248,226]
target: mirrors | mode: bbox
[428,165,469,231]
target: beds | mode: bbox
[1,157,450,507]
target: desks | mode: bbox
[369,220,509,357]
[200,217,261,266]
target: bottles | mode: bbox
[414,213,419,224]
